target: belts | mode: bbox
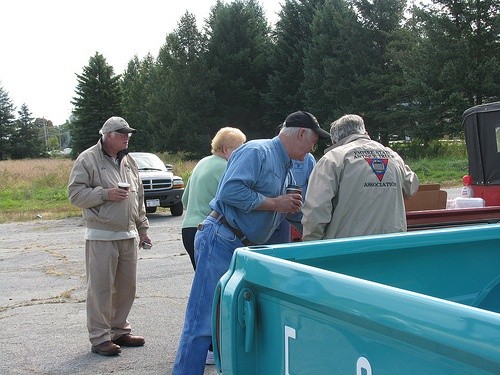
[209,211,249,246]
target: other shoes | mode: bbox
[206,350,216,365]
[91,342,121,356]
[112,333,146,346]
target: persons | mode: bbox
[301,114,419,242]
[291,153,316,204]
[172,111,330,375]
[68,117,153,355]
[181,127,246,364]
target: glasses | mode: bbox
[114,133,132,137]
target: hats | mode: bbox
[283,110,332,141]
[102,117,138,134]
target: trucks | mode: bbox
[210,102,500,375]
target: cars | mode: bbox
[128,152,185,216]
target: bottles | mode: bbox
[460,175,474,198]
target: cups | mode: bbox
[117,182,130,192]
[286,185,302,195]
